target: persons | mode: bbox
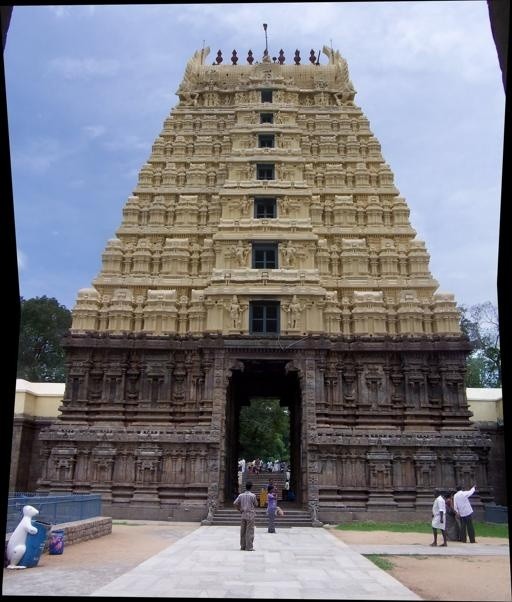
[238,457,290,507]
[234,482,258,551]
[430,490,447,546]
[223,89,307,329]
[445,491,460,541]
[267,486,278,533]
[454,484,477,543]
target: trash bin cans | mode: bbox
[288,490,295,501]
[49,530,64,555]
[6,505,52,569]
